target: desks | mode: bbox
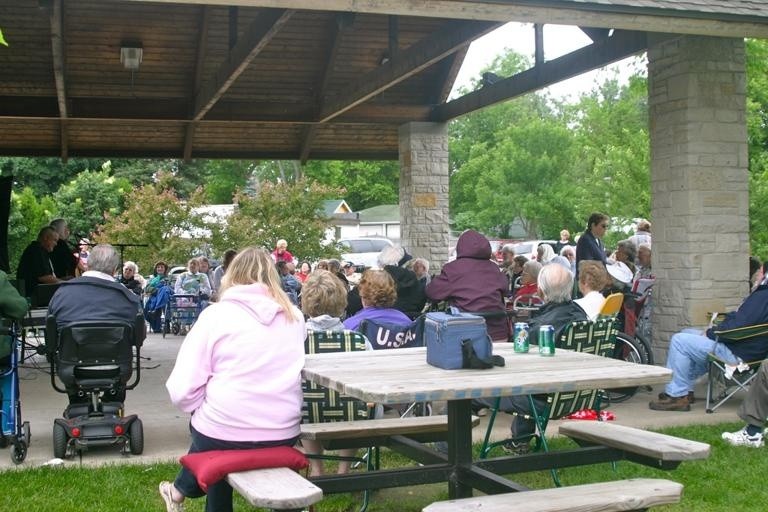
[299,340,674,512]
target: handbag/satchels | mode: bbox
[425,306,487,370]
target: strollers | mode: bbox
[161,293,203,337]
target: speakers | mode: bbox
[31,283,60,307]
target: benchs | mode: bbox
[224,414,713,512]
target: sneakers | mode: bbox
[721,430,764,448]
[649,391,693,411]
[502,442,529,456]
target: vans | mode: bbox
[327,236,395,265]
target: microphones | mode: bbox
[67,231,86,251]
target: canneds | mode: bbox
[538,325,555,356]
[514,322,529,353]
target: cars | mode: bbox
[192,243,224,270]
[449,237,568,267]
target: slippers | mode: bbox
[158,481,182,512]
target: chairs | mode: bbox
[142,287,301,340]
[300,314,426,512]
[476,315,621,485]
[702,312,768,414]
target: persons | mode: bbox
[295,268,374,478]
[0,269,29,364]
[648,263,768,412]
[74,236,91,268]
[142,245,240,336]
[49,217,87,278]
[424,228,510,342]
[155,244,309,512]
[15,226,62,309]
[116,261,143,297]
[269,238,431,319]
[720,358,768,448]
[490,210,652,458]
[46,242,142,406]
[339,266,412,332]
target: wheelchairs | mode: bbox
[515,265,657,410]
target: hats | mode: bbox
[605,261,632,283]
[397,246,412,267]
